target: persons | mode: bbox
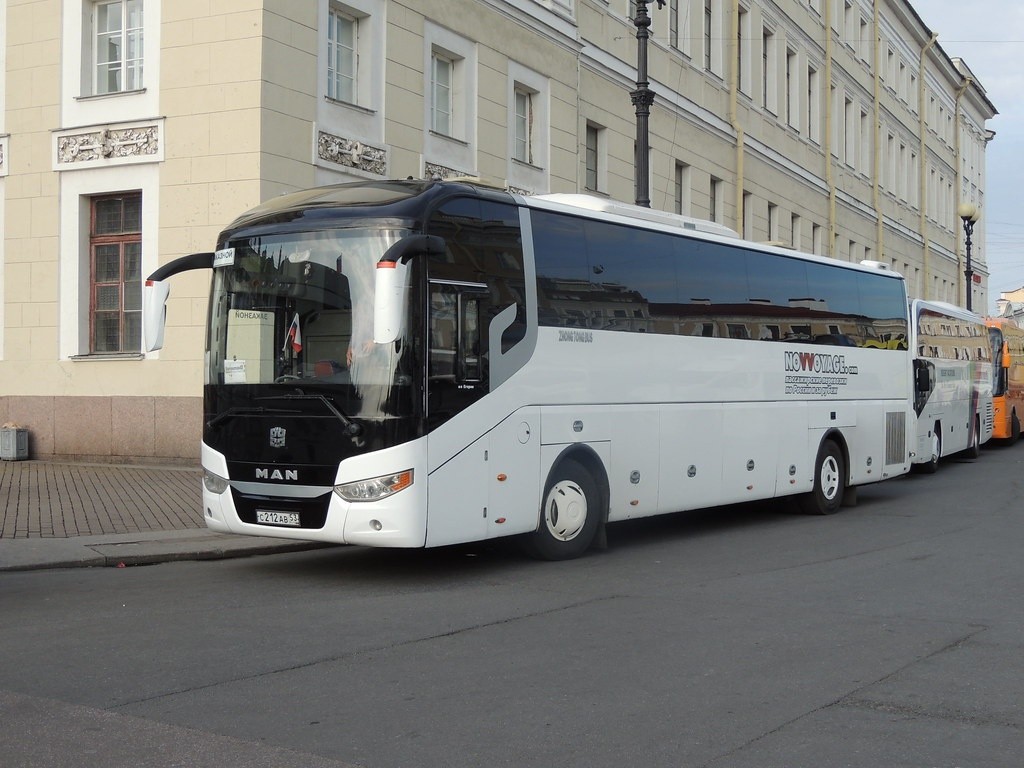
[346,268,379,368]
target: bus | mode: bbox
[146,179,915,563]
[985,316,1024,442]
[904,298,993,476]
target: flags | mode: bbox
[287,313,302,353]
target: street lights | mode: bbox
[959,202,981,313]
